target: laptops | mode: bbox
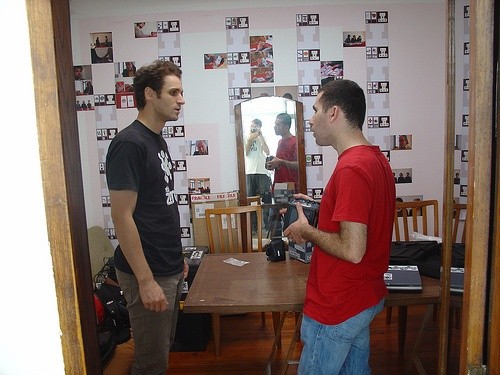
[450,267,465,292]
[382,264,422,290]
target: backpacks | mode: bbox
[412,243,464,280]
[93,256,131,345]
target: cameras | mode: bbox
[251,128,256,132]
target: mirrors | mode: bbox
[235,96,305,255]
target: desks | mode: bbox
[183,254,440,375]
[258,204,294,241]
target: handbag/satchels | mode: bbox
[262,192,273,204]
[266,240,286,261]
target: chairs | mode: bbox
[87,226,115,284]
[204,205,281,355]
[387,201,449,365]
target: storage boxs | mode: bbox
[193,200,240,252]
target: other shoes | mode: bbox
[265,230,271,239]
[252,231,258,235]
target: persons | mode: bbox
[122,62,135,77]
[393,172,412,184]
[266,113,299,194]
[73,66,86,81]
[391,135,412,150]
[243,119,272,197]
[76,100,91,110]
[83,81,93,95]
[345,35,362,44]
[396,197,423,217]
[91,35,113,64]
[193,140,208,155]
[279,81,397,375]
[105,61,189,375]
[134,22,149,38]
